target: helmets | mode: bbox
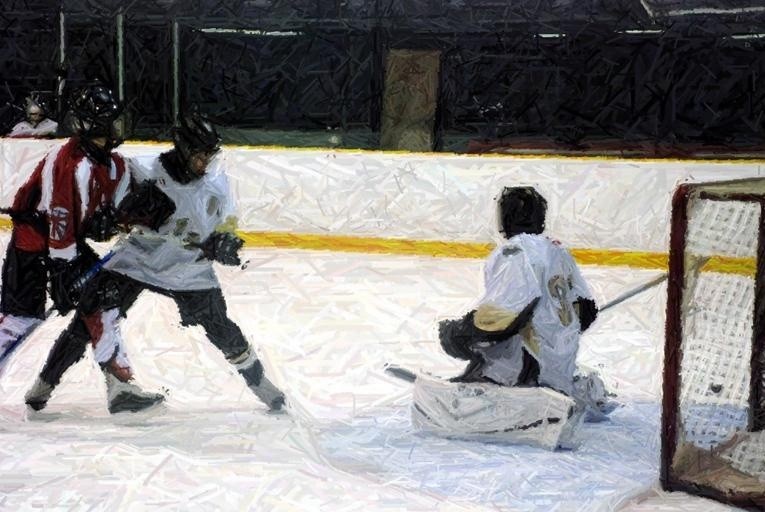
[64,79,129,160]
[495,186,546,235]
[172,111,222,173]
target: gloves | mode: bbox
[50,261,99,316]
[439,316,484,361]
[122,181,175,230]
[88,208,119,243]
[203,231,243,267]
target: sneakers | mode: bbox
[245,372,285,411]
[23,377,55,410]
[106,377,164,415]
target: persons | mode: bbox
[25,114,285,412]
[5,98,59,138]
[441,187,596,449]
[1,84,166,412]
